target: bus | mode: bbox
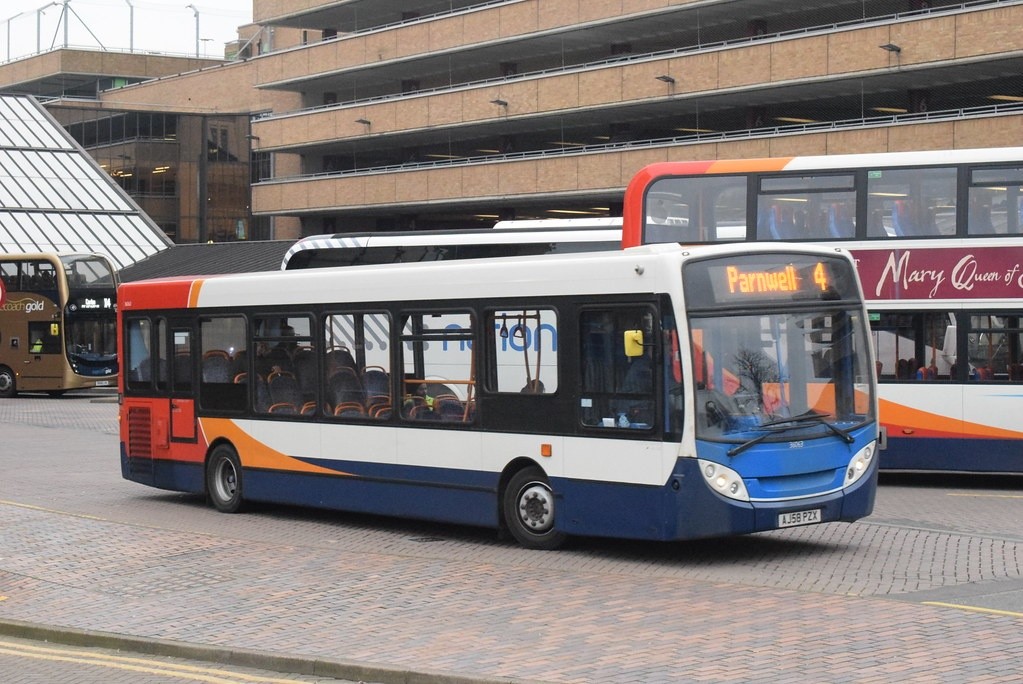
[621,147,1023,478]
[119,241,880,549]
[0,252,119,400]
[280,216,789,402]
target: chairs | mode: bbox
[1,273,88,290]
[788,351,1023,387]
[139,336,459,422]
[767,201,1023,240]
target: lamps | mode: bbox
[354,120,370,125]
[879,44,901,53]
[490,99,508,108]
[654,75,675,84]
[244,134,259,141]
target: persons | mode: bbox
[403,380,436,418]
[251,328,304,378]
[619,336,679,422]
[33,336,47,351]
